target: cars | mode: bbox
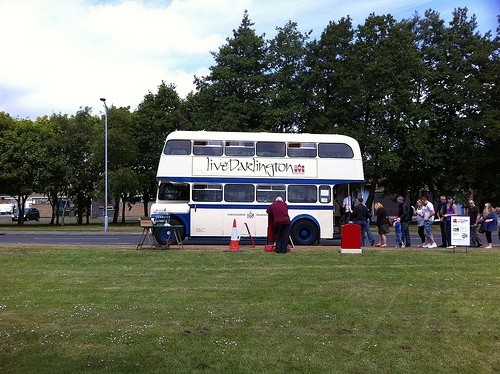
[12,207,40,222]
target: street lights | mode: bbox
[100,98,109,231]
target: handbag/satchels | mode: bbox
[386,218,393,227]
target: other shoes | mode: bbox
[371,239,375,246]
[485,246,492,248]
[282,250,286,253]
[420,243,427,247]
[382,244,387,248]
[422,243,432,248]
[375,243,380,247]
[476,242,483,248]
[401,244,405,248]
[438,244,447,247]
[446,245,456,248]
[276,250,282,253]
[428,244,438,248]
[470,243,476,247]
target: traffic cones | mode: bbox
[228,218,241,251]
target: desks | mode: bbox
[135,225,184,251]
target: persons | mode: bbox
[334,193,374,247]
[395,196,411,247]
[437,195,500,249]
[374,202,389,247]
[413,199,426,247]
[419,197,437,248]
[266,197,290,253]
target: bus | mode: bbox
[150,129,366,246]
